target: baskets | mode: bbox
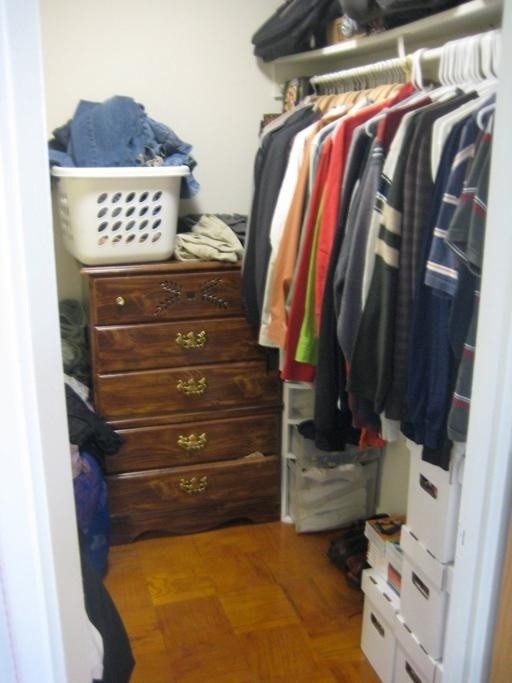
[51,165,191,266]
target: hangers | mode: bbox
[308,26,500,139]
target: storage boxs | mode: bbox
[357,431,466,682]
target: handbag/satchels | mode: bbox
[252,0,332,62]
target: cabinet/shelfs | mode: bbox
[284,382,386,531]
[78,259,284,547]
